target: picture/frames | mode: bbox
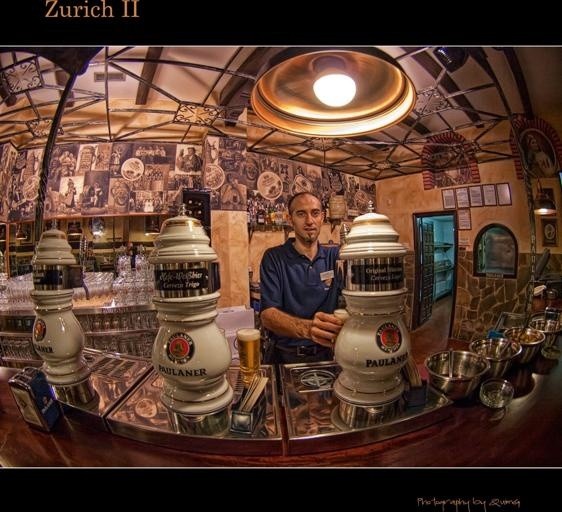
[19,219,81,245]
[143,216,161,236]
[440,184,512,208]
[541,217,557,246]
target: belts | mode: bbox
[274,341,325,356]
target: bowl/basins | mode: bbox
[478,378,514,408]
[502,325,546,366]
[527,311,562,351]
[423,350,490,401]
[468,338,522,381]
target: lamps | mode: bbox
[505,118,557,217]
[249,47,417,141]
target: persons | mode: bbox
[258,191,345,396]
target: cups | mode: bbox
[233,328,262,373]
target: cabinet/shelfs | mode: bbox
[1,305,161,369]
